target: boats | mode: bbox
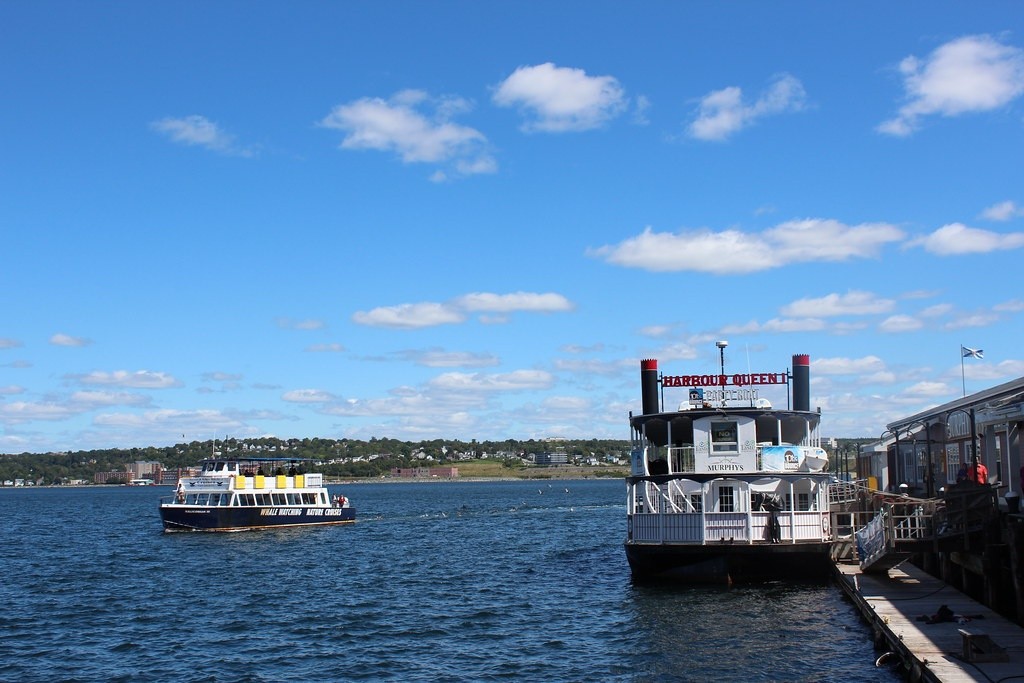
[158,457,356,532]
[624,341,839,584]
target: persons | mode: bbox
[967,457,987,484]
[289,465,297,476]
[177,484,186,504]
[202,463,207,471]
[276,468,283,475]
[957,463,968,480]
[244,470,250,475]
[258,467,264,475]
[331,492,345,507]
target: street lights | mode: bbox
[845,448,848,481]
[907,421,933,498]
[945,408,978,487]
[844,442,875,479]
[880,430,916,485]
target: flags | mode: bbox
[963,347,983,359]
